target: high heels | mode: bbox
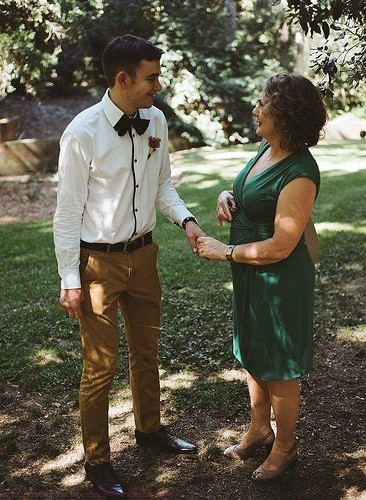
[224,429,276,459]
[251,437,299,483]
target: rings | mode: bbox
[200,249,203,254]
[216,208,219,211]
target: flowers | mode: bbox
[147,135,162,160]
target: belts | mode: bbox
[81,230,153,253]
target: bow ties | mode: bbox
[114,114,150,136]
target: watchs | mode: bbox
[225,245,237,264]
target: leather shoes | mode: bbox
[85,460,124,494]
[136,425,196,453]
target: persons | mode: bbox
[194,74,328,484]
[53,35,208,498]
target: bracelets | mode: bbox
[182,217,197,231]
